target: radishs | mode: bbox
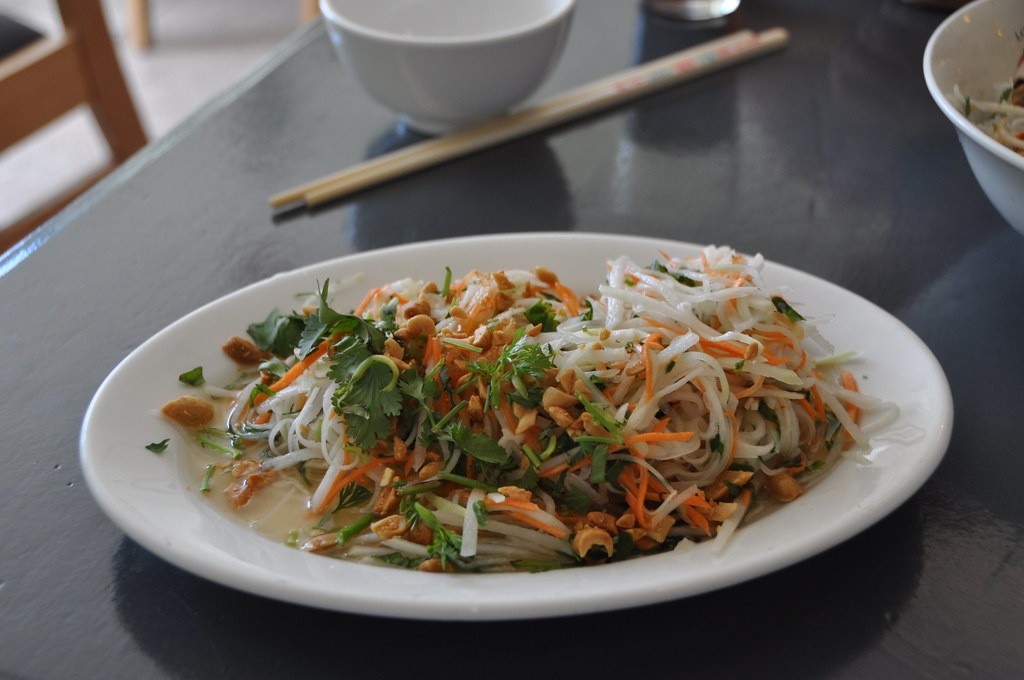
[163,242,902,575]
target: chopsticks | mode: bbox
[265,24,789,218]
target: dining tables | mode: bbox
[1,0,1022,676]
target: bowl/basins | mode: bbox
[316,0,579,136]
[923,0,1024,240]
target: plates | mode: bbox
[76,229,955,623]
